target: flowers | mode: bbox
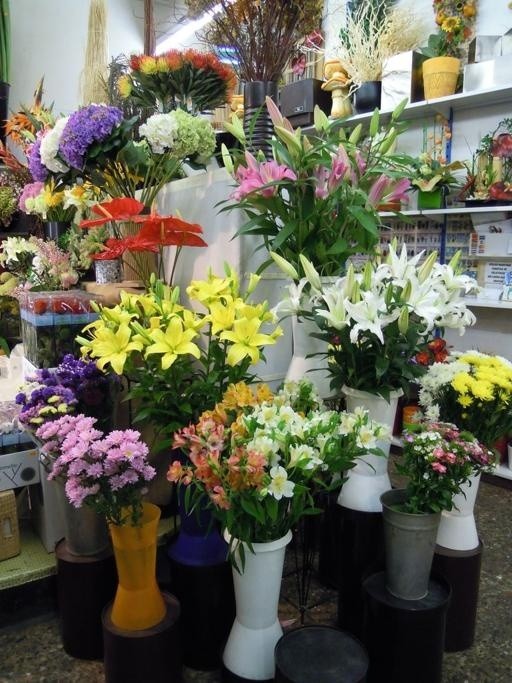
[167,377,389,575]
[16,355,122,457]
[35,412,157,527]
[403,113,470,193]
[269,236,480,406]
[212,95,420,297]
[397,412,495,515]
[418,350,512,451]
[418,0,478,59]
[74,256,284,457]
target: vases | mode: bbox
[222,527,293,680]
[379,489,442,601]
[436,467,482,551]
[353,81,381,114]
[283,275,340,410]
[422,56,460,100]
[106,504,167,630]
[53,456,110,557]
[336,383,403,512]
[243,81,278,163]
[167,452,231,568]
